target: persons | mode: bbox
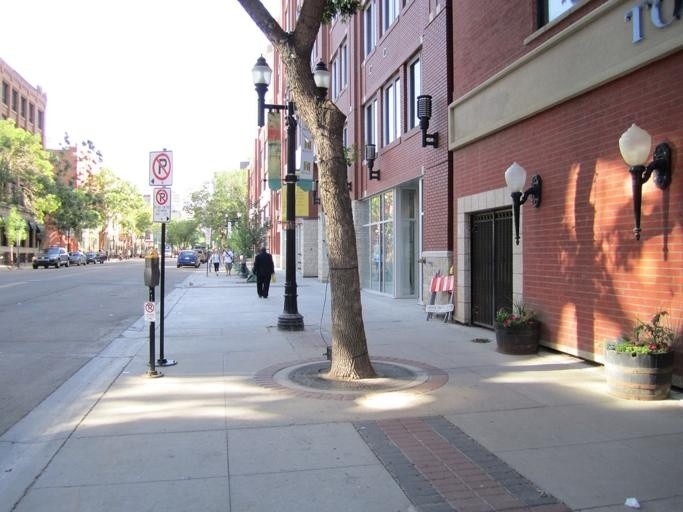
[222,245,233,276]
[211,249,220,276]
[253,247,274,298]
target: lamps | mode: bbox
[619,123,672,240]
[311,181,320,205]
[365,144,380,181]
[417,95,438,148]
[505,161,542,246]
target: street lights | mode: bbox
[223,210,245,279]
[252,53,329,330]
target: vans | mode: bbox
[165,244,172,258]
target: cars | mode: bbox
[31,247,70,268]
[69,251,108,266]
[176,247,214,269]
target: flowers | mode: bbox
[605,311,674,355]
[496,299,537,329]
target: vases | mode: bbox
[604,347,674,400]
[495,320,540,354]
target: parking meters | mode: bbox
[142,245,161,379]
